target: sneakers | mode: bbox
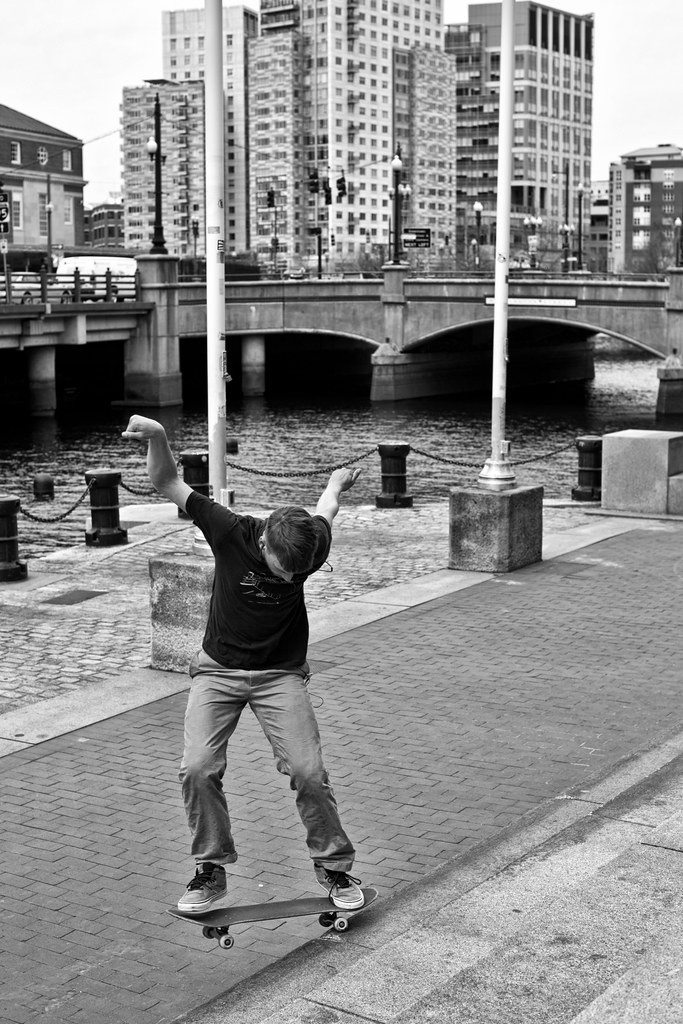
[314,863,364,909]
[178,862,229,914]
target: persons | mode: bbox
[121,415,367,911]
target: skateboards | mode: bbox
[164,886,380,949]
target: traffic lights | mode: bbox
[267,190,274,207]
[296,242,300,253]
[331,235,335,246]
[310,179,319,192]
[336,179,347,197]
[366,232,370,243]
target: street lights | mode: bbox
[524,216,542,270]
[557,224,574,273]
[552,171,568,274]
[391,155,412,263]
[577,183,585,269]
[147,137,168,253]
[675,216,682,268]
[473,200,484,271]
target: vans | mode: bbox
[55,256,137,302]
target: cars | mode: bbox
[0,273,72,304]
[342,272,378,279]
